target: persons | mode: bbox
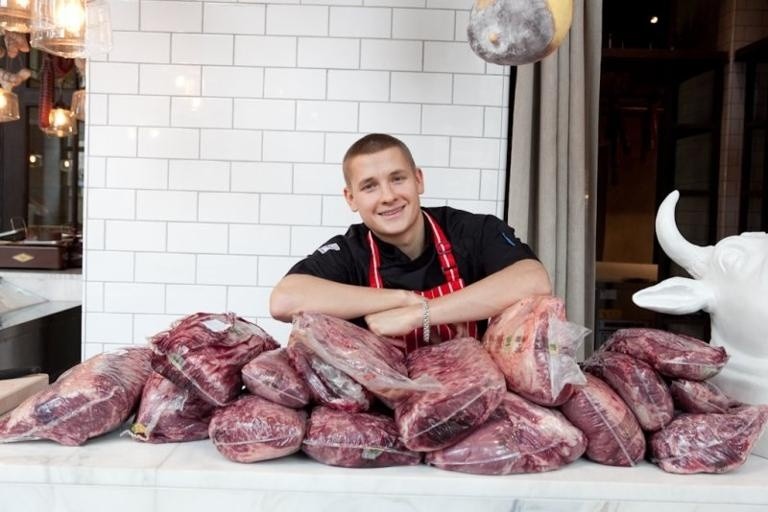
[269,134,553,356]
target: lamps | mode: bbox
[28,0,115,59]
[0,88,21,123]
[71,86,86,122]
[39,82,78,138]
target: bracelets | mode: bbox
[420,299,431,343]
